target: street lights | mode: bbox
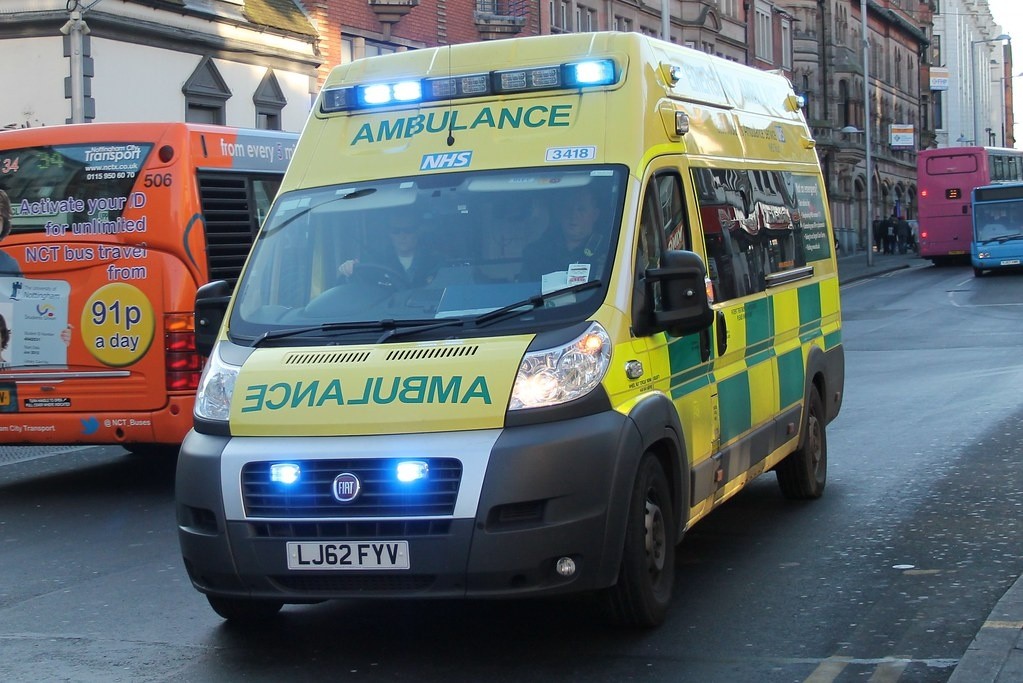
[842,126,873,267]
[985,128,991,146]
[956,35,1011,146]
[991,133,996,147]
[1000,73,1023,148]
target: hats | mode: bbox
[900,216,905,220]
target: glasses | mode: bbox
[387,224,419,235]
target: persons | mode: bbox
[521,192,608,281]
[872,215,912,254]
[339,209,446,290]
[0,188,73,362]
[0,314,11,362]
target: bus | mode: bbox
[0,121,310,454]
[971,183,1023,277]
[916,146,1023,266]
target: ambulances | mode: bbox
[177,31,844,628]
